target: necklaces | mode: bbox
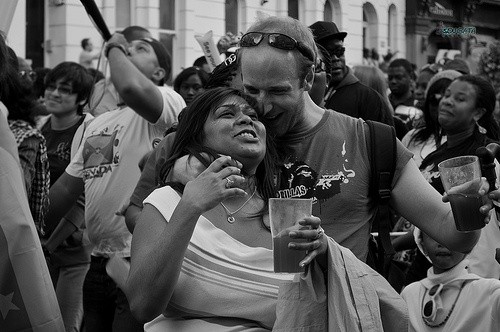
[422,281,466,328]
[117,102,126,106]
[220,186,257,224]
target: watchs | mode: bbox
[105,42,128,58]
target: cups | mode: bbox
[268,198,312,274]
[438,156,488,234]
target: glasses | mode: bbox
[45,84,71,95]
[420,63,438,73]
[325,46,346,58]
[423,282,445,321]
[18,70,37,79]
[239,31,297,51]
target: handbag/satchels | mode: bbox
[366,118,432,292]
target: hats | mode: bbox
[309,20,347,43]
[16,56,32,71]
[217,32,240,53]
[141,36,173,85]
[442,58,469,74]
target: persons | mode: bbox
[0,0,500,332]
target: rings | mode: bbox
[225,177,234,187]
[311,228,321,239]
